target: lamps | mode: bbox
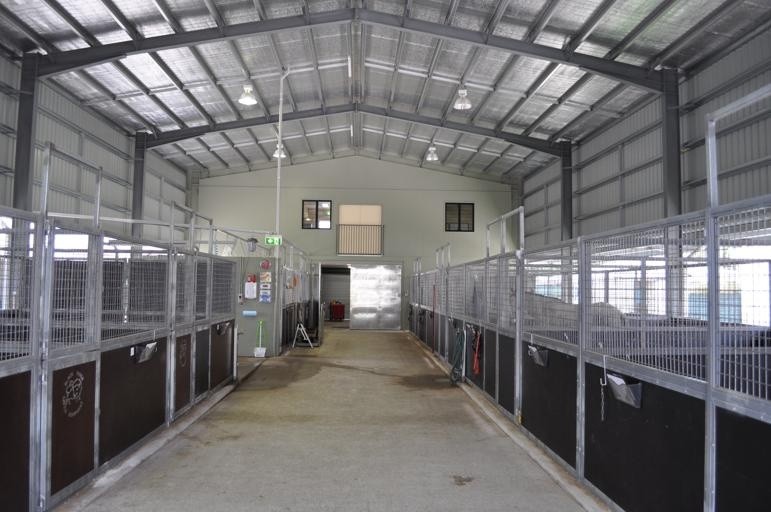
[237,79,258,106]
[425,143,439,162]
[273,143,287,158]
[454,79,471,110]
